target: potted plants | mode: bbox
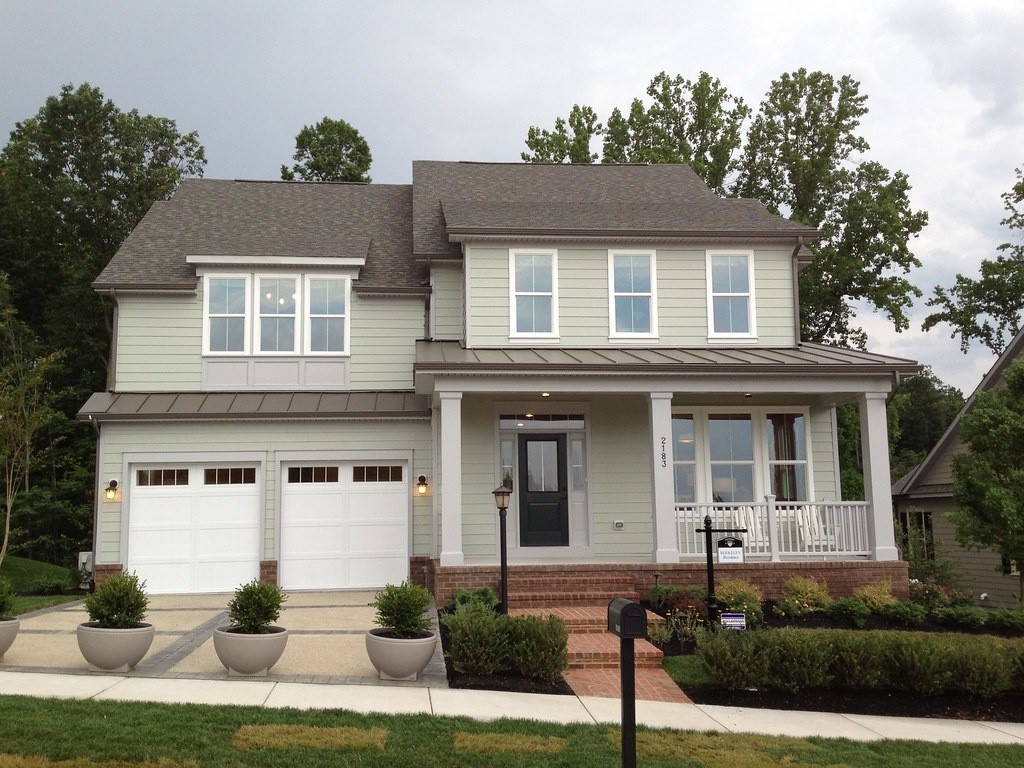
[76,567,154,672]
[366,577,437,681]
[213,576,289,676]
[0,575,21,658]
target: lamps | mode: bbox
[491,483,512,509]
[417,476,428,494]
[105,481,118,499]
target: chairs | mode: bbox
[735,507,771,547]
[797,506,839,551]
